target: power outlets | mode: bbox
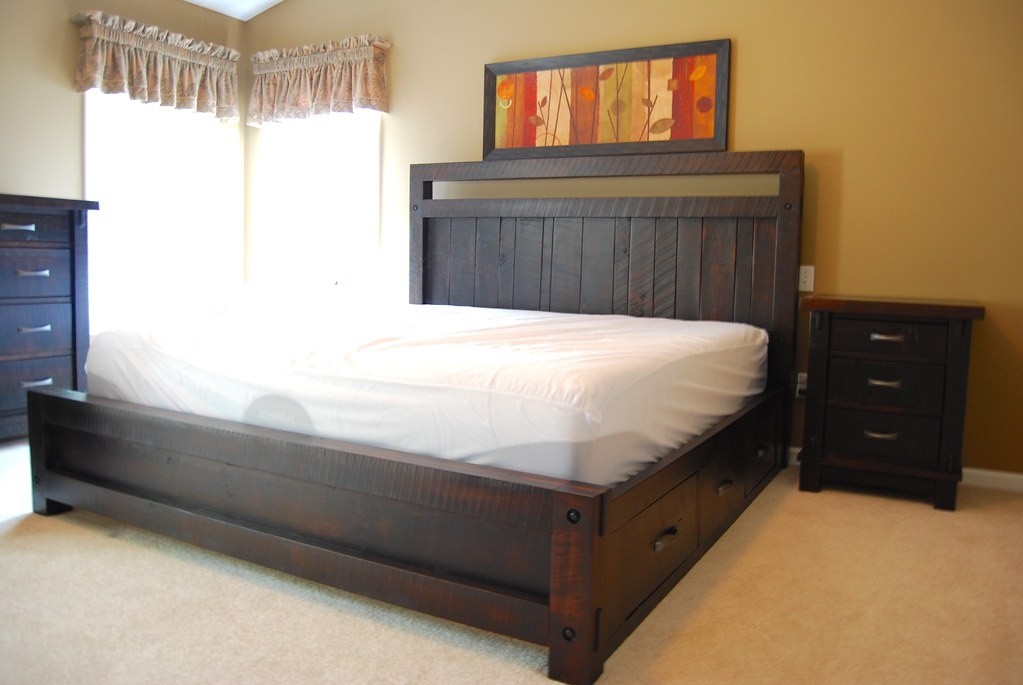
[796,373,807,397]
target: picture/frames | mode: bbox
[482,37,732,160]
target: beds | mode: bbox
[24,144,804,685]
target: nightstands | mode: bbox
[795,292,985,517]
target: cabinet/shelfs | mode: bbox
[0,194,99,441]
